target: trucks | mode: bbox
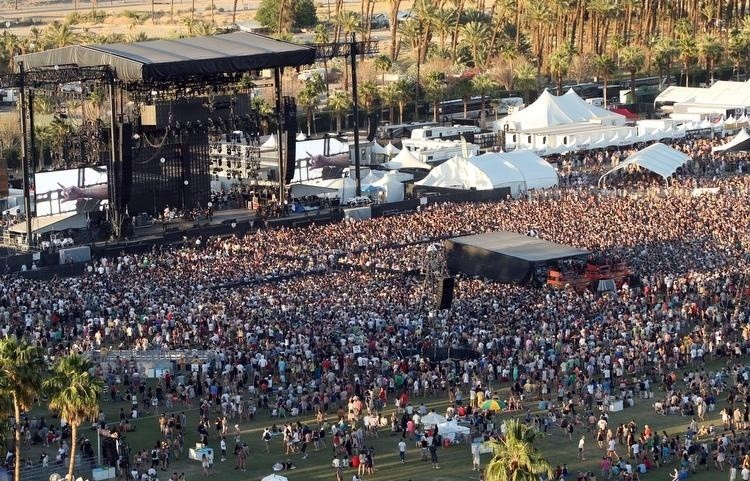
[2,87,21,105]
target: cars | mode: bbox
[397,12,417,28]
[359,13,385,29]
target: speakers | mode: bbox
[344,207,371,219]
[6,253,33,269]
[436,277,455,310]
[58,246,91,265]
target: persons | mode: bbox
[0,131,750,481]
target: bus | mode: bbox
[376,120,452,147]
[627,74,678,91]
[410,125,481,140]
[549,81,598,99]
[438,96,494,122]
